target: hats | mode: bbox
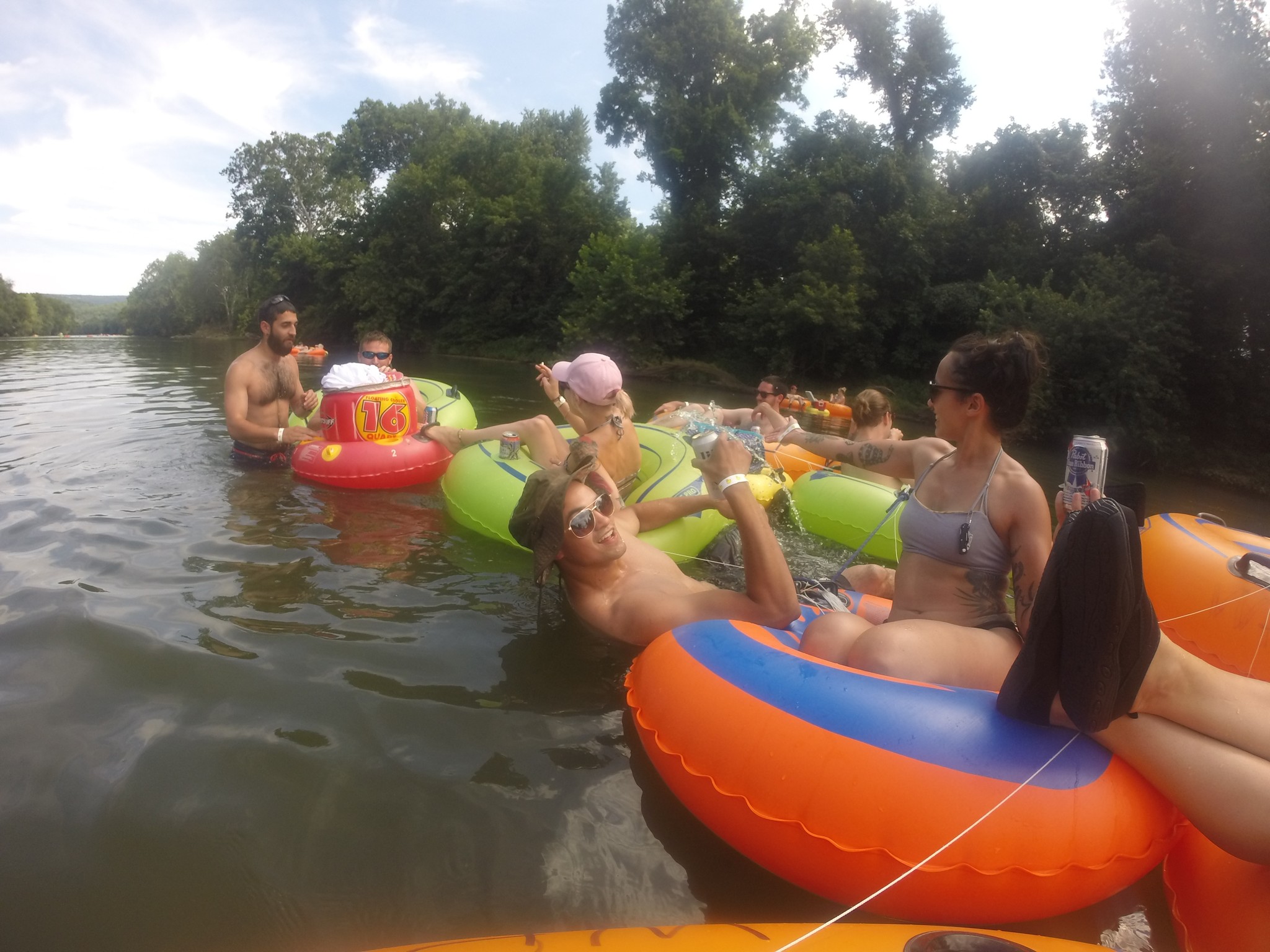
[790,385,797,389]
[551,353,622,406]
[508,454,597,588]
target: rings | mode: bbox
[558,461,562,465]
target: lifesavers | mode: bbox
[290,422,456,489]
[1138,513,1270,684]
[288,376,478,446]
[804,405,830,417]
[623,588,1189,923]
[780,399,811,411]
[647,414,842,478]
[441,422,737,565]
[366,925,1117,952]
[747,466,794,508]
[1165,819,1270,952]
[789,469,911,561]
[819,400,852,419]
[291,348,329,356]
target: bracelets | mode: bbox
[553,396,566,407]
[719,474,749,492]
[779,423,801,443]
[278,427,284,442]
[685,401,689,406]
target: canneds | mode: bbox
[499,431,520,460]
[690,431,728,502]
[1062,434,1109,514]
[423,406,438,425]
[750,426,761,434]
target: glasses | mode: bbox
[559,380,588,404]
[563,492,614,539]
[929,374,978,397]
[362,351,392,360]
[264,295,290,310]
[754,388,776,398]
[883,410,895,423]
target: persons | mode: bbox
[508,431,895,645]
[419,352,641,495]
[752,332,1106,692]
[997,498,1270,863]
[293,343,328,355]
[786,386,808,409]
[650,375,789,433]
[830,387,846,405]
[840,389,915,492]
[224,299,321,462]
[307,331,427,430]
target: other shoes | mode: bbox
[1055,496,1161,734]
[995,509,1082,727]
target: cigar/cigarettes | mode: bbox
[541,362,544,370]
